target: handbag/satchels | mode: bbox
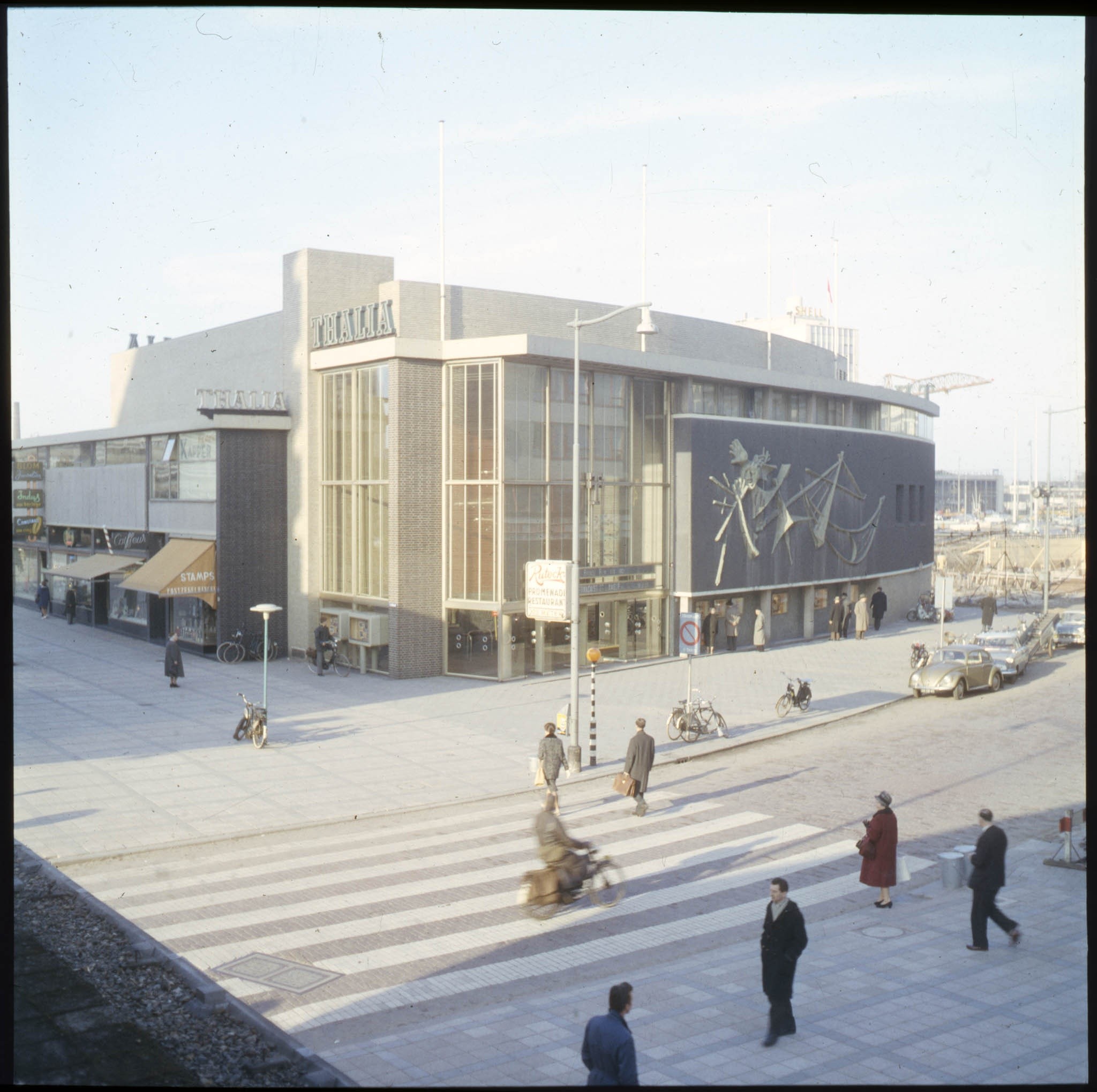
[534,758,545,787]
[34,594,40,604]
[612,773,635,797]
[855,834,868,849]
[859,837,875,862]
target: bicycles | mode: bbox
[303,639,352,677]
[665,690,729,743]
[910,642,929,669]
[904,587,956,622]
[216,623,280,665]
[233,694,271,749]
[774,671,812,717]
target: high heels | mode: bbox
[874,899,892,908]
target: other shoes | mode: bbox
[170,683,180,688]
[763,1033,778,1048]
[42,615,48,619]
[570,892,583,900]
[966,945,989,951]
[727,648,733,651]
[632,803,649,817]
[318,673,325,676]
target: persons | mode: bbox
[538,723,568,815]
[64,583,77,625]
[829,586,888,642]
[164,631,185,688]
[760,878,808,1046]
[724,601,742,651]
[535,795,593,905]
[624,719,655,817]
[315,617,338,677]
[966,810,1022,951]
[859,792,898,909]
[980,591,998,634]
[753,608,767,652]
[702,608,719,655]
[35,582,51,620]
[581,982,641,1087]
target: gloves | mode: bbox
[862,819,870,828]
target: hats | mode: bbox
[726,597,733,605]
[833,596,840,600]
[859,593,868,600]
[874,790,892,808]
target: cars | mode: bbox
[973,630,1030,683]
[1053,611,1085,650]
[911,644,1004,700]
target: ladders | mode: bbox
[1022,611,1061,656]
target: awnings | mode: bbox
[42,554,144,580]
[114,538,218,611]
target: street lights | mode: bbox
[565,305,657,773]
[249,604,284,723]
